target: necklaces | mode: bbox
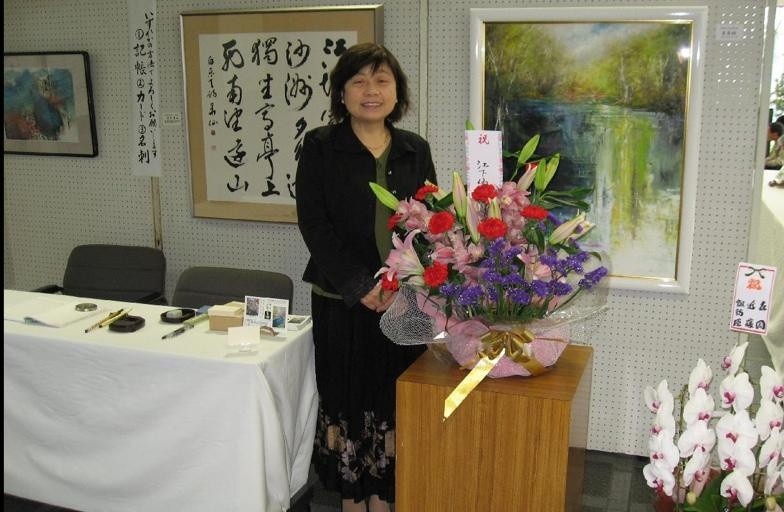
[354,124,392,151]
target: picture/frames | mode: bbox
[177,2,384,225]
[468,4,711,294]
[4,49,98,159]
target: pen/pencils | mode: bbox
[162,324,195,340]
[83,308,135,334]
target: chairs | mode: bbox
[36,243,168,303]
[172,268,292,318]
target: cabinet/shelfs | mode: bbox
[391,341,599,510]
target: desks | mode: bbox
[3,288,316,512]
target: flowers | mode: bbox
[365,124,608,327]
[642,344,783,512]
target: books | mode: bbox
[2,293,108,327]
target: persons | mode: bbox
[763,117,784,187]
[295,43,440,510]
